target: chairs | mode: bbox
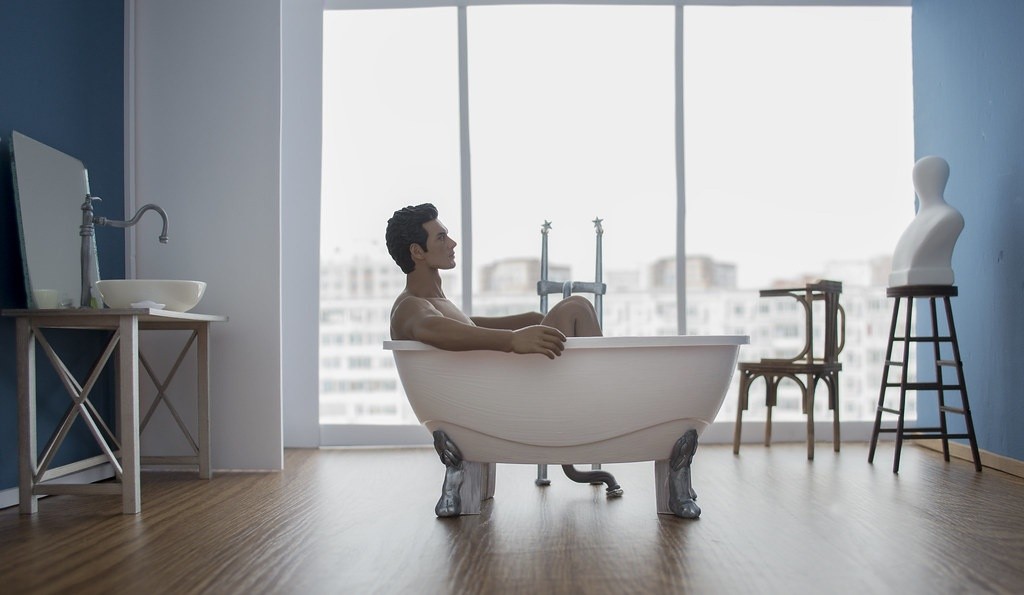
[733,279,845,461]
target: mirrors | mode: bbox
[8,129,105,308]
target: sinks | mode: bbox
[95,278,207,312]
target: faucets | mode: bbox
[80,192,170,307]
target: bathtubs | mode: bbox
[382,335,755,518]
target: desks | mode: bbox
[5,308,230,515]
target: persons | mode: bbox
[386,203,604,359]
[890,156,966,285]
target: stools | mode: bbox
[868,286,983,475]
